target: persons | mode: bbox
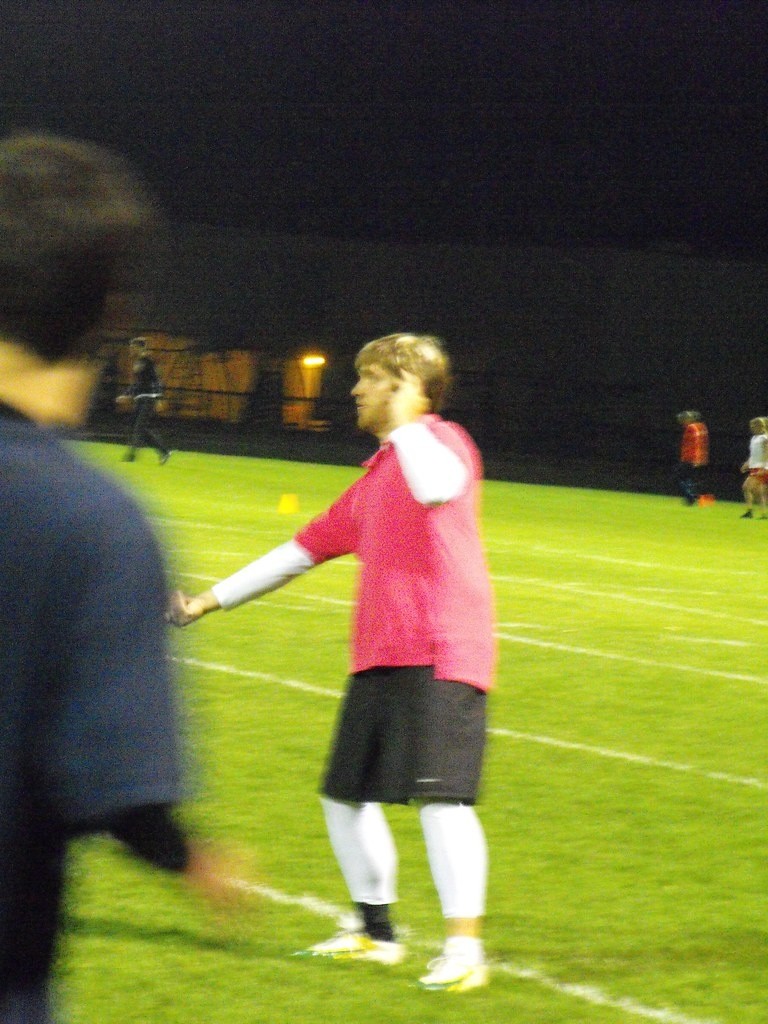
[739,417,768,520]
[165,333,495,991]
[116,338,172,466]
[0,136,198,1024]
[674,411,709,505]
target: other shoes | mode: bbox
[740,512,753,518]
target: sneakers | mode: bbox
[305,916,403,969]
[409,936,489,995]
